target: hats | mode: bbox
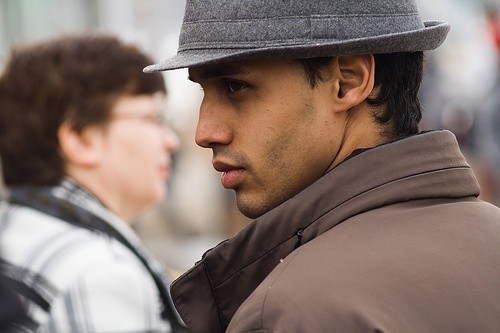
[143,0,451,72]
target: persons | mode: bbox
[0,34,187,333]
[143,0,500,333]
